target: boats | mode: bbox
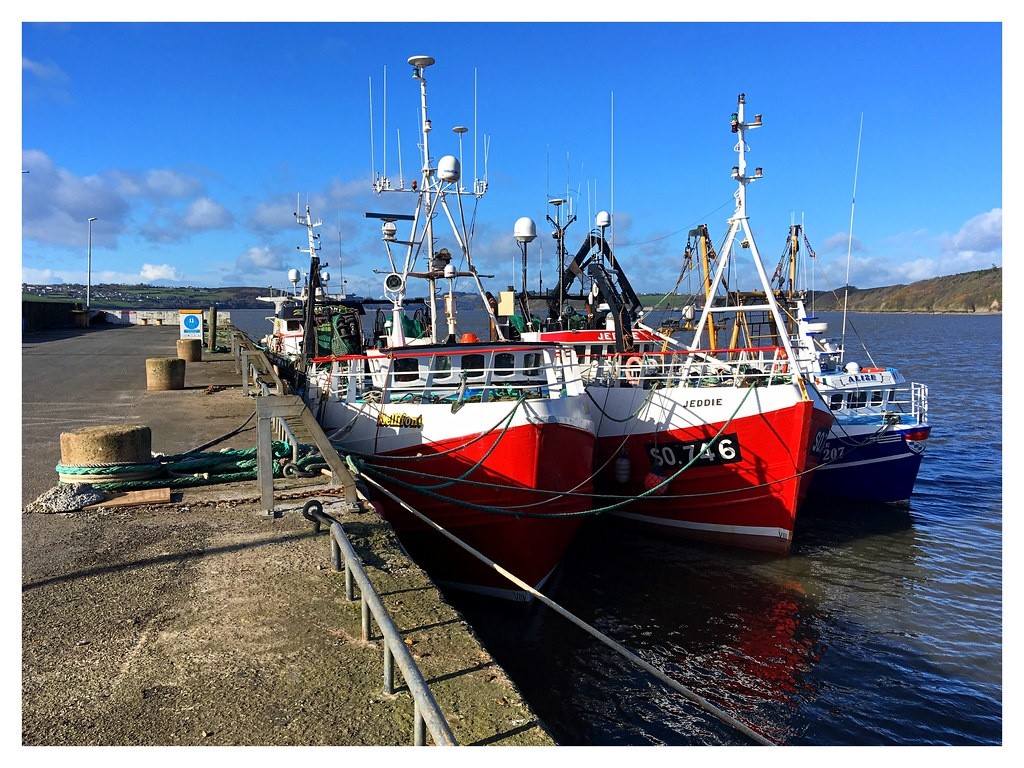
[257,54,595,604]
[653,109,932,513]
[492,89,835,557]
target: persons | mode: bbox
[485,291,498,342]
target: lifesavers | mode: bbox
[774,350,789,375]
[625,355,644,386]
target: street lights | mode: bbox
[87,217,98,307]
